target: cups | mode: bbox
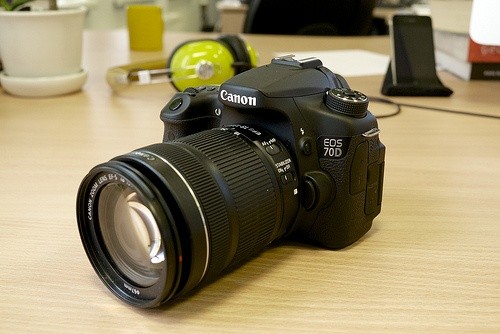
[127,4,163,51]
[219,4,248,33]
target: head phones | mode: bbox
[106,34,259,92]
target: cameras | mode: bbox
[76,53,386,309]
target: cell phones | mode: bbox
[391,15,437,86]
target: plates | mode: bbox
[0,72,88,97]
